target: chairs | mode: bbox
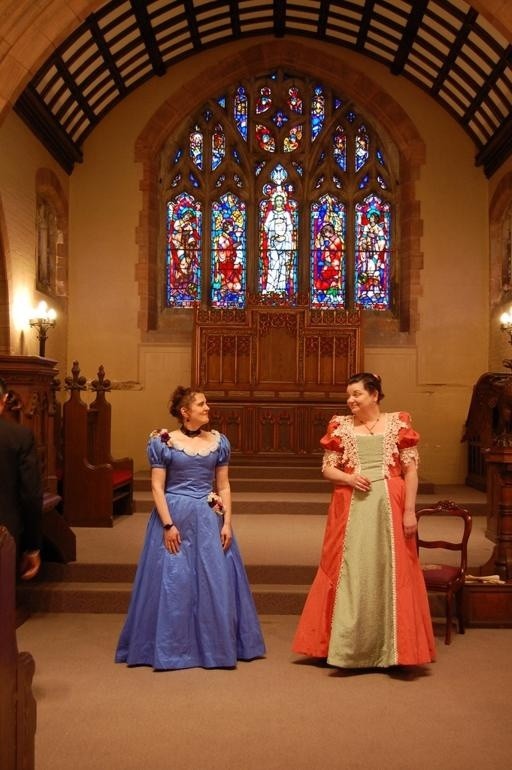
[414,500,473,646]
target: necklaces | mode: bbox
[180,424,201,437]
[358,417,380,436]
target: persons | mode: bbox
[142,386,232,671]
[0,377,44,583]
[318,371,420,676]
[262,194,294,294]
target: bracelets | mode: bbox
[161,523,175,530]
